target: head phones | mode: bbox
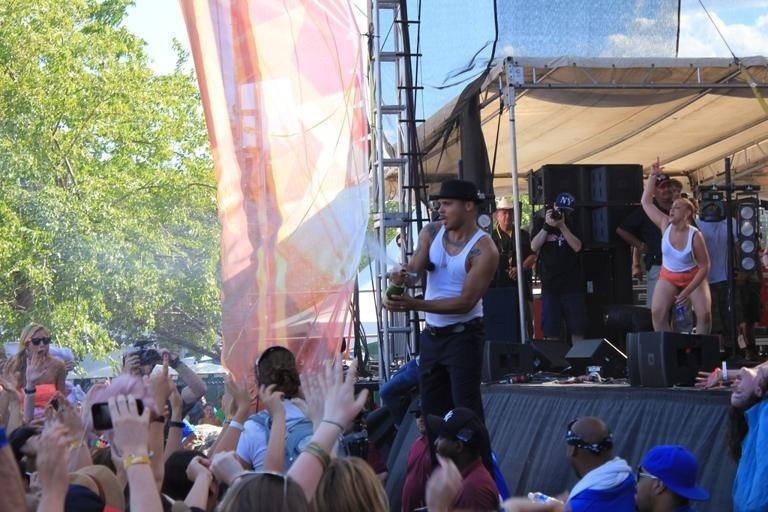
[255,345,292,391]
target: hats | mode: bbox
[556,193,576,213]
[65,483,124,512]
[429,180,497,201]
[426,407,487,448]
[638,444,712,502]
[655,174,673,188]
[68,465,126,512]
[496,199,513,209]
[409,400,422,414]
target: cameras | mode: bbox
[122,340,160,365]
[550,209,562,220]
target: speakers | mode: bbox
[482,340,534,385]
[735,190,761,273]
[565,338,624,379]
[528,339,571,374]
[626,331,721,387]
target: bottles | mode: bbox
[528,492,562,503]
[382,269,407,305]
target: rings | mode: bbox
[400,305,404,311]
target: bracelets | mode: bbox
[170,421,185,428]
[304,443,331,469]
[25,388,36,394]
[154,416,165,422]
[224,420,231,424]
[230,421,244,431]
[321,419,345,430]
[123,454,150,469]
[70,439,86,450]
[170,357,181,368]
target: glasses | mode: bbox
[28,337,50,346]
[632,463,661,483]
[414,412,423,418]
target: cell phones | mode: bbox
[90,398,145,430]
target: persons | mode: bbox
[425,452,464,511]
[120,341,206,436]
[546,416,636,510]
[616,175,693,332]
[1,351,387,512]
[695,363,768,460]
[402,407,430,510]
[641,156,712,335]
[491,197,536,336]
[428,406,503,512]
[731,206,763,361]
[669,174,682,197]
[633,444,711,511]
[532,192,585,344]
[693,187,740,354]
[382,179,498,475]
